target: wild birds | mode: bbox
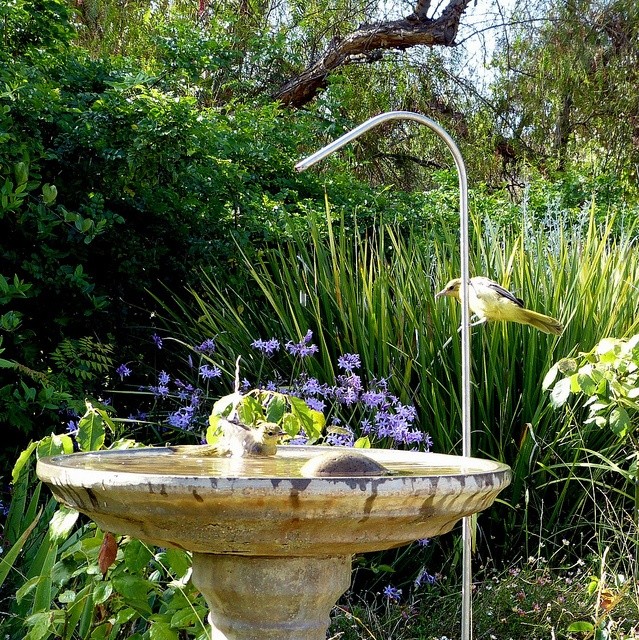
[223,419,288,460]
[436,276,564,336]
